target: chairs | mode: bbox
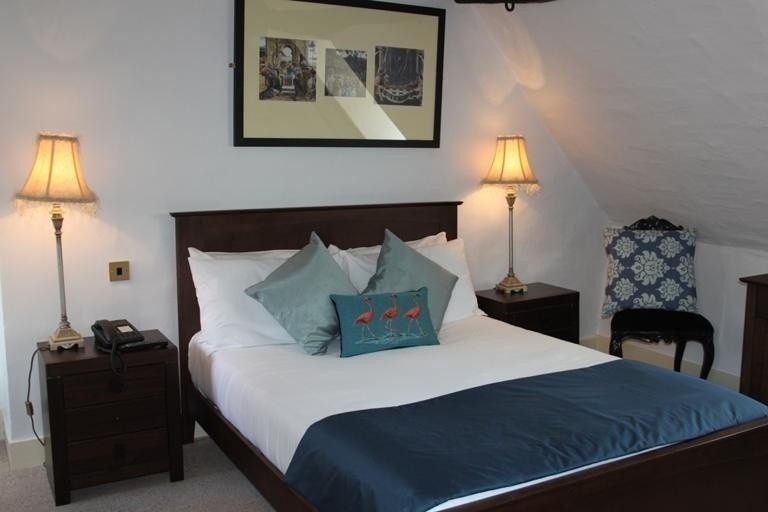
[609,215,714,379]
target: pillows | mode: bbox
[328,286,440,360]
[333,231,486,327]
[243,233,359,355]
[360,228,458,338]
[601,226,696,318]
[188,245,350,339]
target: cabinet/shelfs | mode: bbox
[740,283,768,405]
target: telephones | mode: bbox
[91,319,145,349]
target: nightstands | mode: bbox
[37,329,184,505]
[475,282,579,345]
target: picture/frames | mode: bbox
[234,0,446,147]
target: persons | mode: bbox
[259,50,423,102]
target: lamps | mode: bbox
[481,135,541,296]
[15,133,99,351]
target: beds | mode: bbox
[169,201,768,512]
